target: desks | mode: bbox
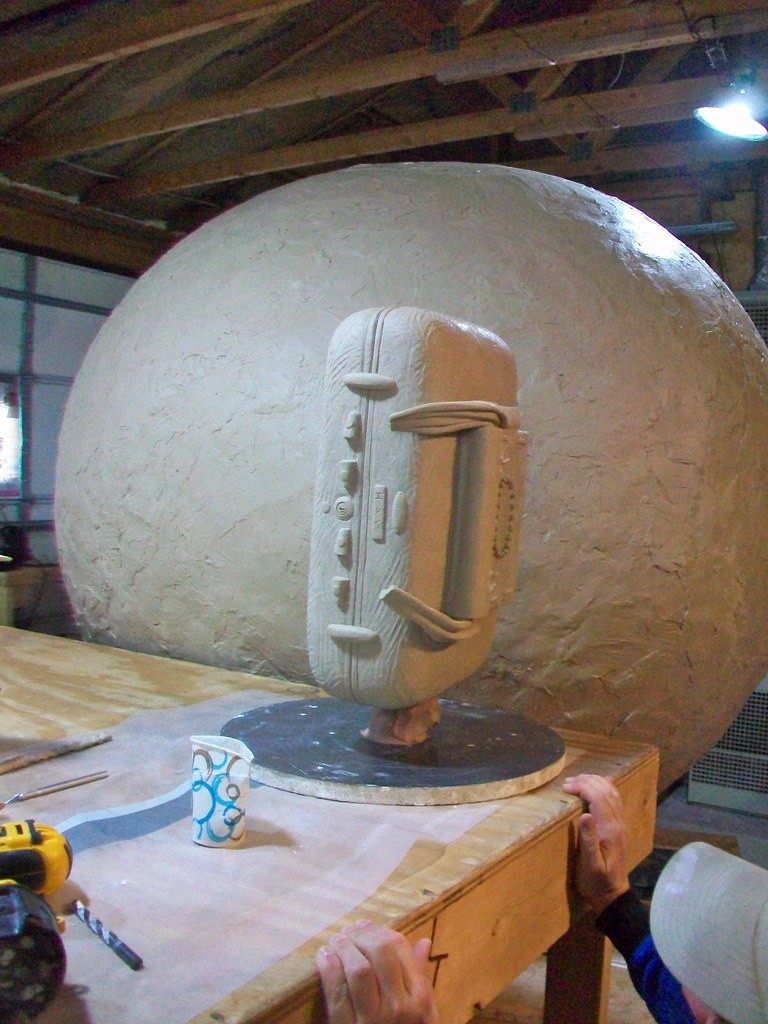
[2,625,659,1024]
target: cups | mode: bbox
[190,735,253,848]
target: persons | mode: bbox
[313,770,768,1024]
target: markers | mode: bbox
[70,898,144,970]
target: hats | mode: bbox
[649,840,768,1024]
